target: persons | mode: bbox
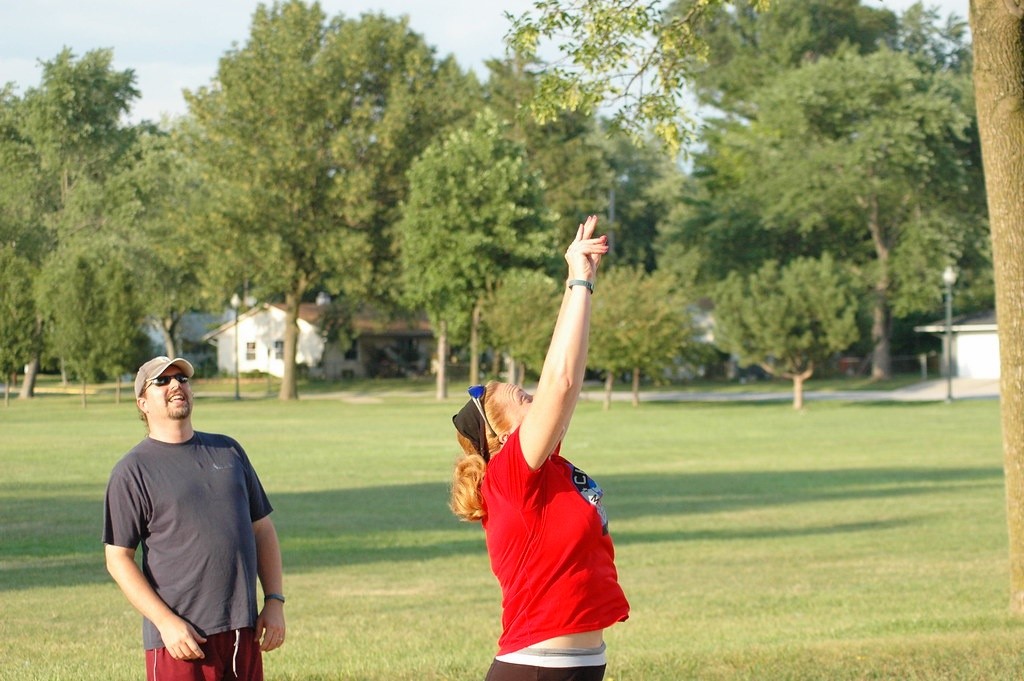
[449,215,630,681]
[102,356,285,681]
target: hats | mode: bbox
[134,355,194,400]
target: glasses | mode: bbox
[468,385,500,444]
[141,373,189,397]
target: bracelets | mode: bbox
[568,280,594,295]
[264,594,285,603]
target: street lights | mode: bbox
[230,293,243,398]
[943,265,958,400]
